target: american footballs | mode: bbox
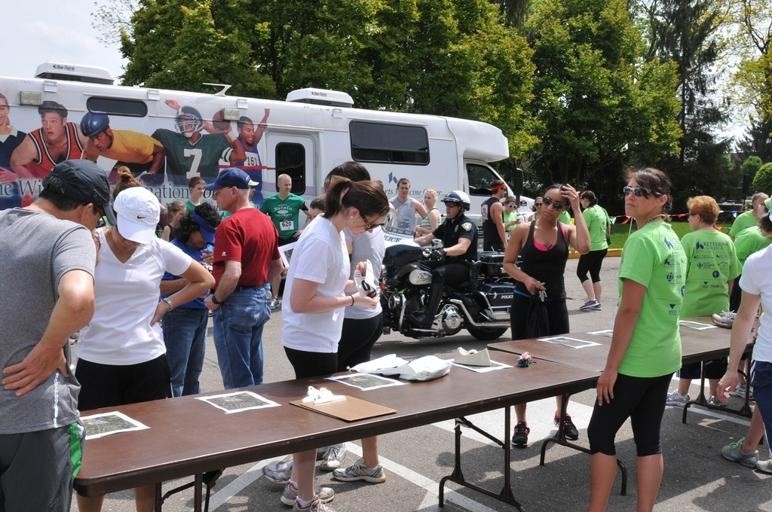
[213,110,231,131]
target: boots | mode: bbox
[410,279,446,329]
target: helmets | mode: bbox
[440,190,471,211]
[80,111,110,137]
[176,105,204,132]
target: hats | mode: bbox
[204,167,251,191]
[51,159,117,226]
[248,178,260,186]
[113,186,162,246]
[191,206,216,243]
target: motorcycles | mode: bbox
[378,236,524,347]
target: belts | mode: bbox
[209,284,255,293]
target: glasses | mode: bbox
[506,204,517,209]
[622,186,654,197]
[445,201,461,208]
[359,211,378,231]
[542,198,562,210]
[535,202,543,207]
[82,201,107,229]
[213,186,231,196]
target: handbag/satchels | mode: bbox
[600,206,611,246]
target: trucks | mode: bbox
[2,61,537,261]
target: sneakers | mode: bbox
[332,457,387,484]
[665,392,692,408]
[291,495,336,512]
[710,394,727,406]
[754,458,772,474]
[580,299,603,312]
[281,480,336,506]
[719,438,759,468]
[313,443,346,471]
[262,454,294,485]
[512,421,530,447]
[554,411,578,440]
[728,383,756,401]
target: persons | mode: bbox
[382,178,521,330]
[0,95,269,204]
[0,160,218,510]
[201,168,313,391]
[665,193,772,472]
[586,168,690,512]
[263,161,388,512]
[501,181,612,446]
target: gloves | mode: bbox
[428,249,447,266]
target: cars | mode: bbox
[613,200,752,226]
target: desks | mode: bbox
[487,315,757,498]
[72,348,627,511]
